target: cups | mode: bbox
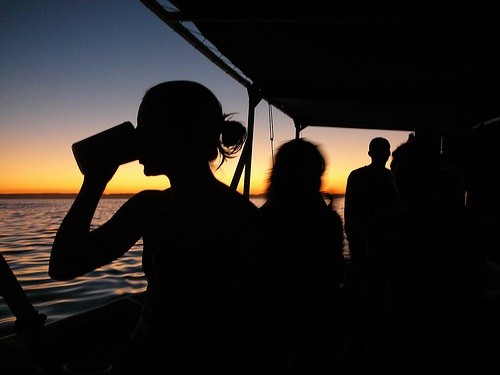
[70,121,141,175]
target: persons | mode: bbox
[259,136,345,261]
[373,131,500,375]
[47,77,277,304]
[342,136,398,259]
